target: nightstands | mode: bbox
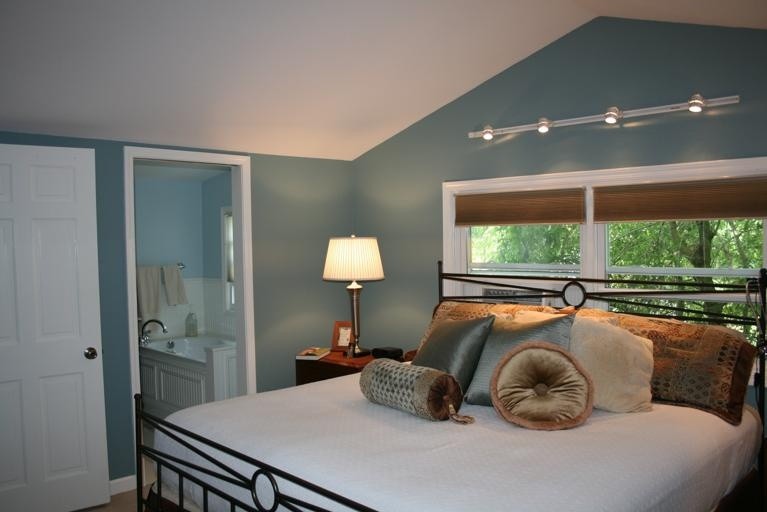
[296,352,400,385]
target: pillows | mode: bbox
[574,308,760,426]
[419,299,574,355]
[464,312,574,406]
[360,358,475,424]
[412,315,495,395]
[489,341,594,430]
[569,316,654,410]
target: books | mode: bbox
[294,345,331,361]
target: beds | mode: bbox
[134,260,766,512]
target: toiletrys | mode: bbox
[185,304,198,337]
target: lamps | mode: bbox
[323,235,386,358]
[468,93,739,140]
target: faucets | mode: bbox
[139,319,169,345]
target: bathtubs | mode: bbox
[140,331,238,408]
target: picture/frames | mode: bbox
[331,321,353,352]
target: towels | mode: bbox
[162,264,187,306]
[136,265,162,313]
